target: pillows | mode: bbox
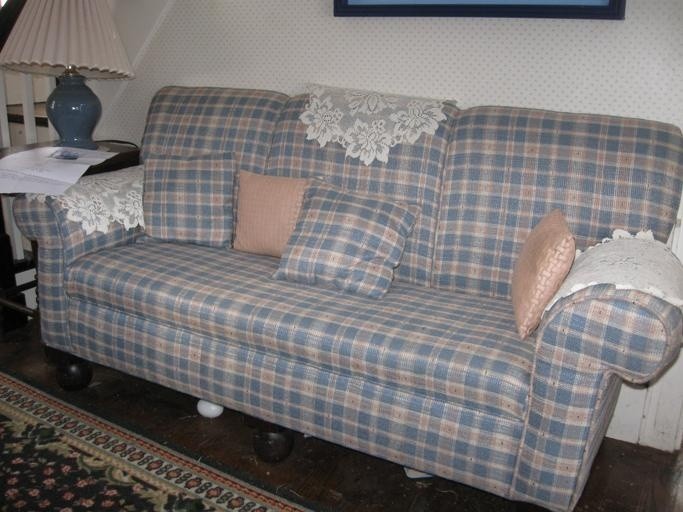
[142,151,240,250]
[511,208,577,343]
[232,169,315,259]
[270,177,421,301]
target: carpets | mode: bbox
[0,367,321,510]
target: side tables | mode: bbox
[1,140,139,331]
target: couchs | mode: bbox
[11,86,682,511]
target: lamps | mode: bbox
[1,1,138,152]
